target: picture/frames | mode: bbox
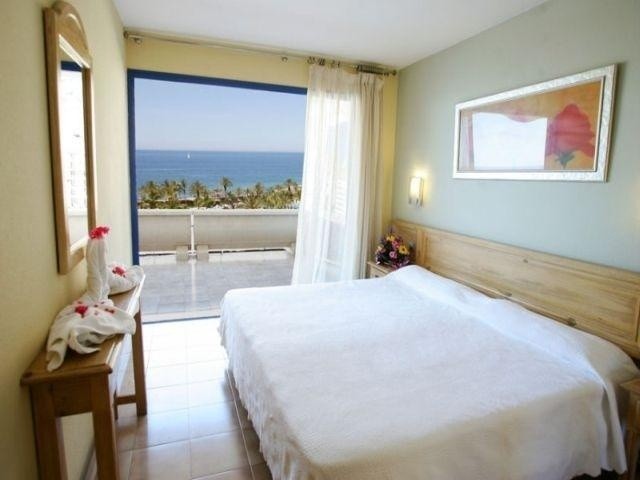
[452,63,617,184]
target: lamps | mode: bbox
[408,176,426,209]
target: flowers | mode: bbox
[374,227,414,270]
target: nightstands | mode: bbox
[619,377,640,480]
[365,254,410,279]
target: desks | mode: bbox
[20,266,146,480]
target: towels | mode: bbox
[42,224,144,372]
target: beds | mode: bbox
[217,263,640,480]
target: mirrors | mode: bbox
[43,0,101,277]
